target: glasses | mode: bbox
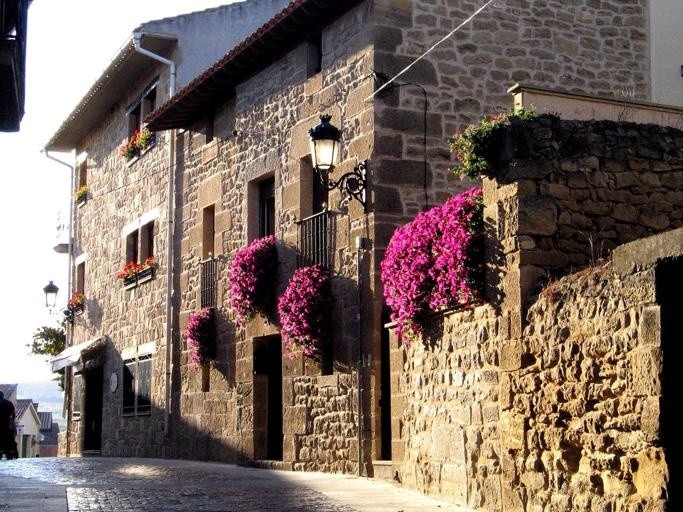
[40,276,66,318]
[306,114,369,213]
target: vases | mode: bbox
[65,291,84,314]
[74,184,87,201]
[116,128,153,158]
[115,257,159,285]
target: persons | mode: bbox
[0,390,17,460]
